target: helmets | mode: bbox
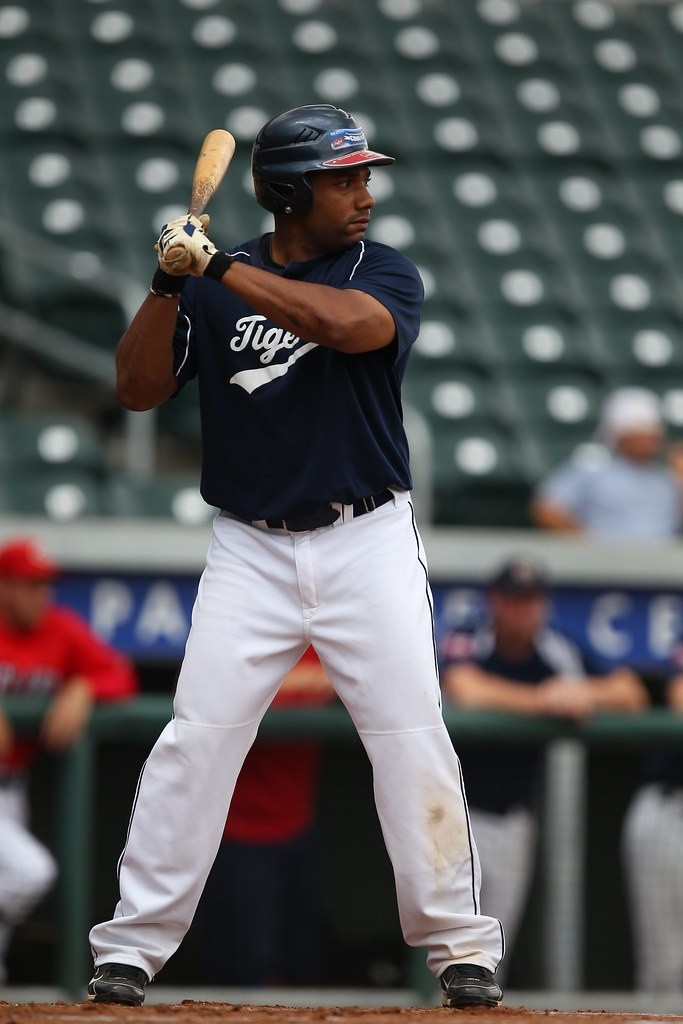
[251,104,394,217]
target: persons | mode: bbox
[442,558,594,994]
[2,538,138,989]
[82,102,505,1010]
[193,642,341,989]
[532,384,683,541]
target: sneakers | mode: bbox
[440,964,504,1007]
[87,962,149,1007]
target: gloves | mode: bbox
[151,213,238,290]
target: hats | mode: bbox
[485,556,560,594]
[0,533,63,582]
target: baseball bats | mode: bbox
[161,125,237,273]
